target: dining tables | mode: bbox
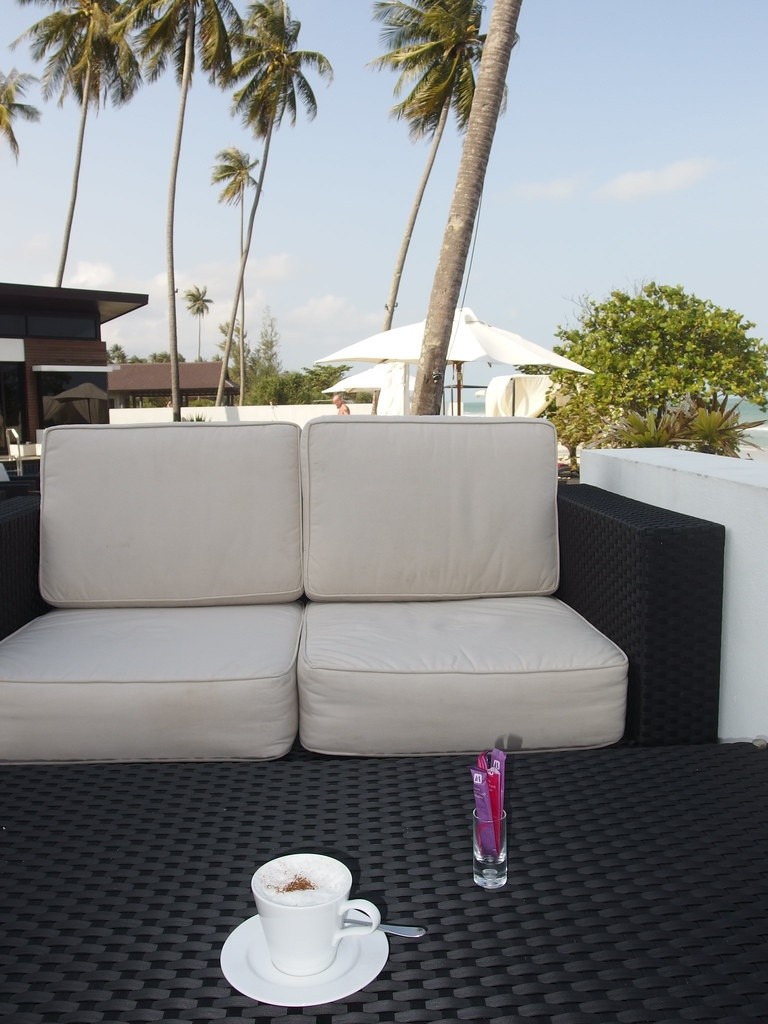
[0,741,768,1024]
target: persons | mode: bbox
[332,395,351,415]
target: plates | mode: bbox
[221,909,388,1006]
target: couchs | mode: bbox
[0,420,725,762]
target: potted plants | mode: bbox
[514,277,767,486]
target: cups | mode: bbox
[251,854,380,977]
[473,807,508,888]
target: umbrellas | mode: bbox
[314,306,596,413]
